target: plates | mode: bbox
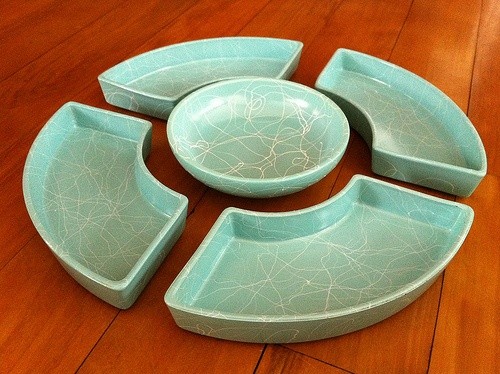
[95,36,305,121]
[161,174,474,344]
[314,47,487,200]
[21,102,189,309]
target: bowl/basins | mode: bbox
[165,77,350,198]
